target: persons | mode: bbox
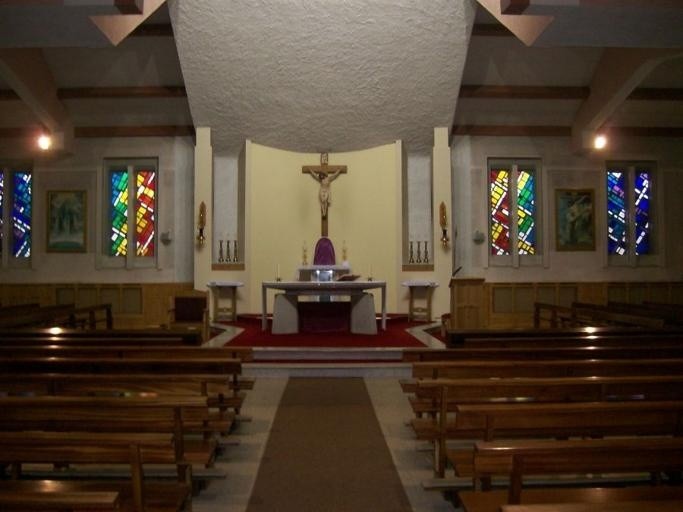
[566,194,590,234]
[308,166,344,219]
[56,197,78,240]
[565,206,587,244]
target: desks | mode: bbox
[258,278,389,332]
[400,276,441,324]
[205,278,245,327]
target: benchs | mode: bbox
[391,298,681,507]
[0,299,258,512]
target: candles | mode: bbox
[217,231,222,241]
[438,199,448,227]
[417,231,422,242]
[223,230,229,242]
[423,232,429,242]
[196,200,206,228]
[367,261,370,277]
[409,231,415,242]
[231,230,238,241]
[275,263,281,276]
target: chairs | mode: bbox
[161,287,211,344]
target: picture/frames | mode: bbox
[43,187,88,254]
[553,186,596,251]
